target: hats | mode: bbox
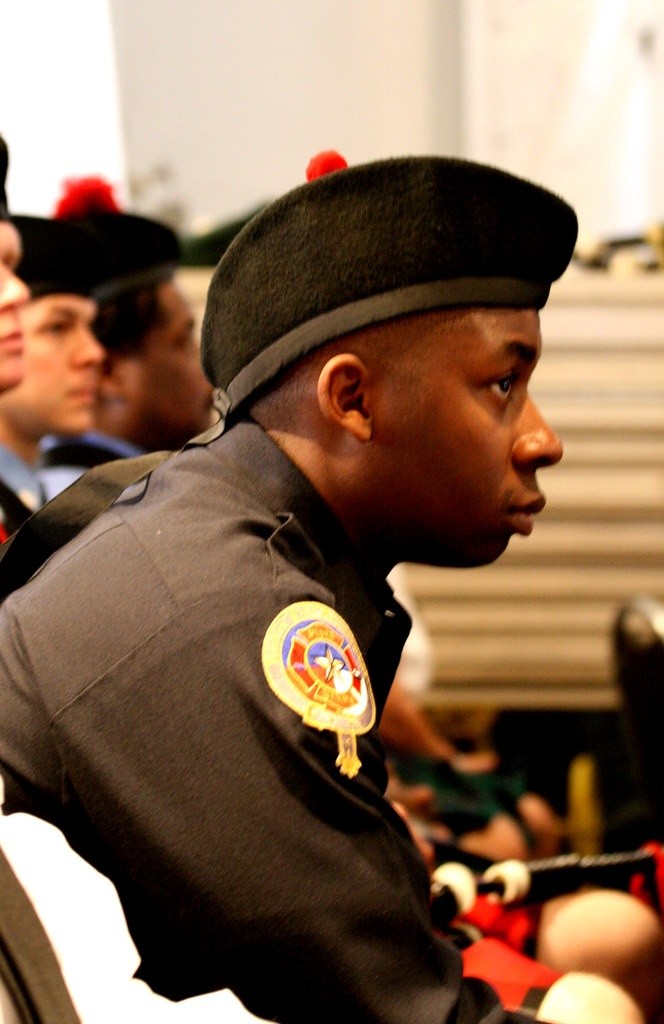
[0,135,15,222]
[51,177,179,303]
[199,148,578,425]
[11,215,97,300]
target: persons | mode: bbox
[0,133,664,1024]
